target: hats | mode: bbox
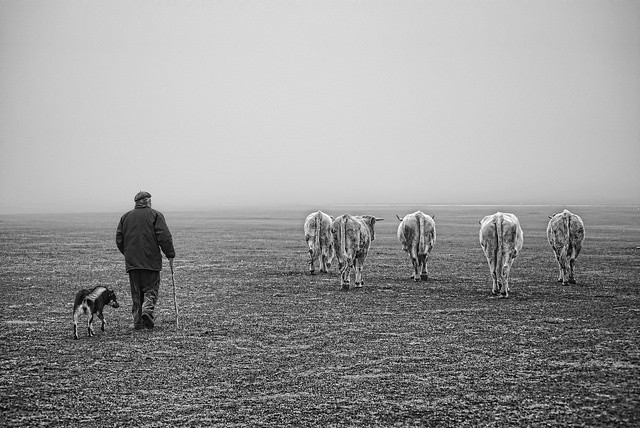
[133,189,153,201]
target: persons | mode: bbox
[116,192,176,329]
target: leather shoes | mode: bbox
[140,312,154,330]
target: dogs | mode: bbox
[73,286,119,340]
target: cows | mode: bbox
[479,212,523,299]
[304,210,336,275]
[396,211,436,282]
[331,213,384,291]
[547,210,585,286]
